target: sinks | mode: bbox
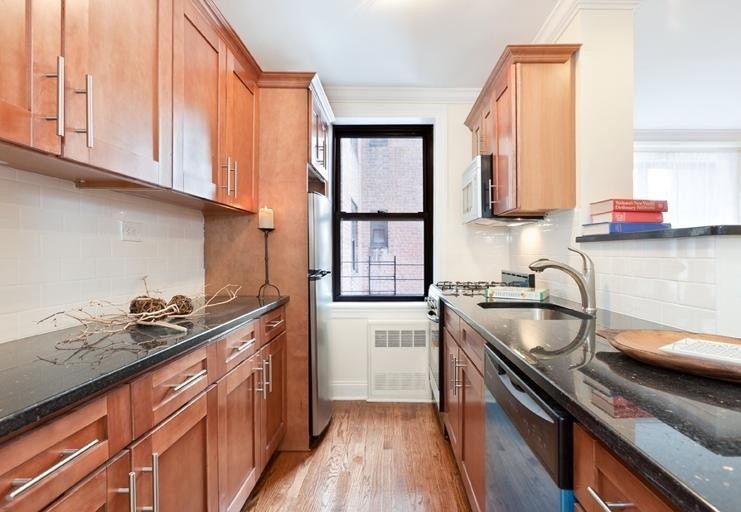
[476,301,595,320]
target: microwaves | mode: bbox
[460,154,543,228]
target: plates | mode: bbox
[596,327,741,386]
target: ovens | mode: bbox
[427,294,441,409]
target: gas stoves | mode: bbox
[432,281,514,297]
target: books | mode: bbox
[591,211,663,223]
[582,222,671,236]
[575,365,657,418]
[589,199,668,216]
[484,285,551,301]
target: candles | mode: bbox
[259,205,273,228]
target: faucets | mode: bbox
[526,248,598,311]
[528,321,598,371]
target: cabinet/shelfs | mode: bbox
[0,0,174,190]
[570,420,681,512]
[489,43,583,217]
[173,0,263,217]
[217,302,290,512]
[463,78,498,161]
[0,339,218,512]
[442,304,486,512]
[258,72,336,183]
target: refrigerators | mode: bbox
[308,189,333,439]
